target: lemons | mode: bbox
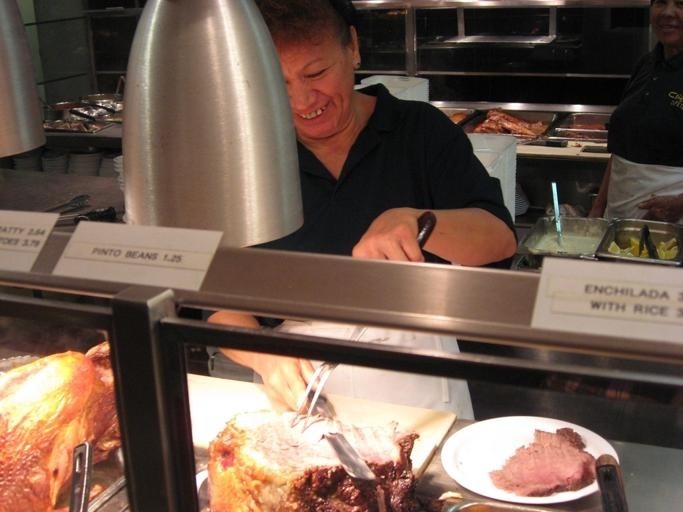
[607,237,673,260]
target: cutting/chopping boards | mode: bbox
[185,371,459,488]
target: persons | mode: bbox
[588,0,683,224]
[194,2,520,421]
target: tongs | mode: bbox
[25,190,117,226]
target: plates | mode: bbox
[14,149,124,179]
[439,415,621,505]
